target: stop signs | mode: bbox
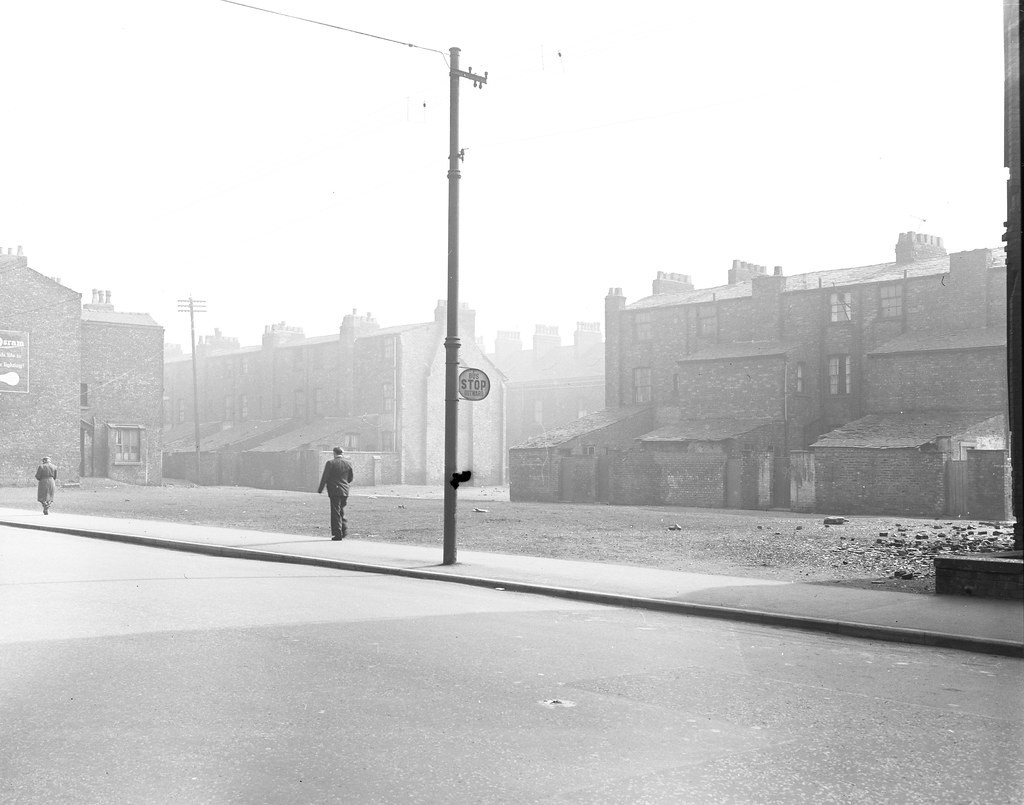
[459,368,491,402]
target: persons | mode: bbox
[34,457,57,516]
[317,447,353,542]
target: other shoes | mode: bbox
[343,530,347,538]
[332,536,342,540]
